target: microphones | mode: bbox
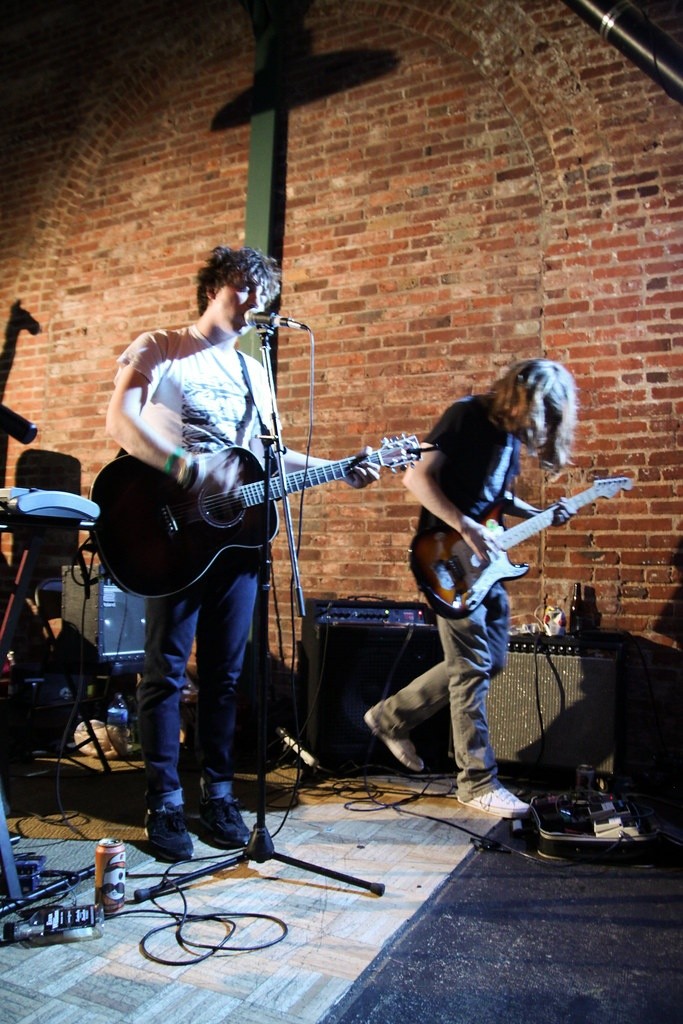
[244,308,309,331]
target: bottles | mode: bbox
[0,903,105,947]
[8,668,19,700]
[107,694,128,760]
[125,696,142,760]
[569,582,587,638]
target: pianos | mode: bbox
[0,486,100,521]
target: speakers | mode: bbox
[61,564,149,663]
[486,633,629,788]
[298,598,450,776]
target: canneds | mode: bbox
[94,839,125,911]
[544,610,566,637]
[576,765,595,789]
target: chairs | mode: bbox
[26,575,148,773]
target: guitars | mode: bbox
[88,431,440,600]
[408,474,633,621]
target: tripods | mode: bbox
[131,327,386,903]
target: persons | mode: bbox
[365,358,576,818]
[107,246,381,862]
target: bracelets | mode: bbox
[163,447,185,472]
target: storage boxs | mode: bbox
[61,565,148,662]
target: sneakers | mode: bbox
[199,792,252,848]
[144,804,193,861]
[457,782,531,819]
[363,707,425,773]
